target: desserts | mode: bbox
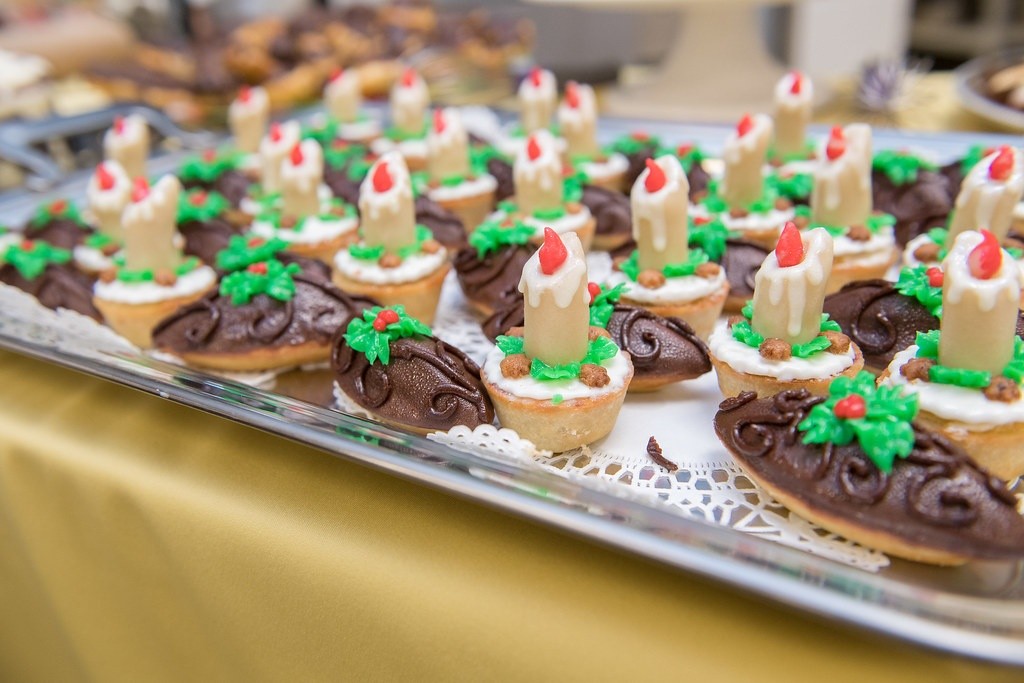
[4,64,1024,569]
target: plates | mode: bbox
[946,40,1024,132]
